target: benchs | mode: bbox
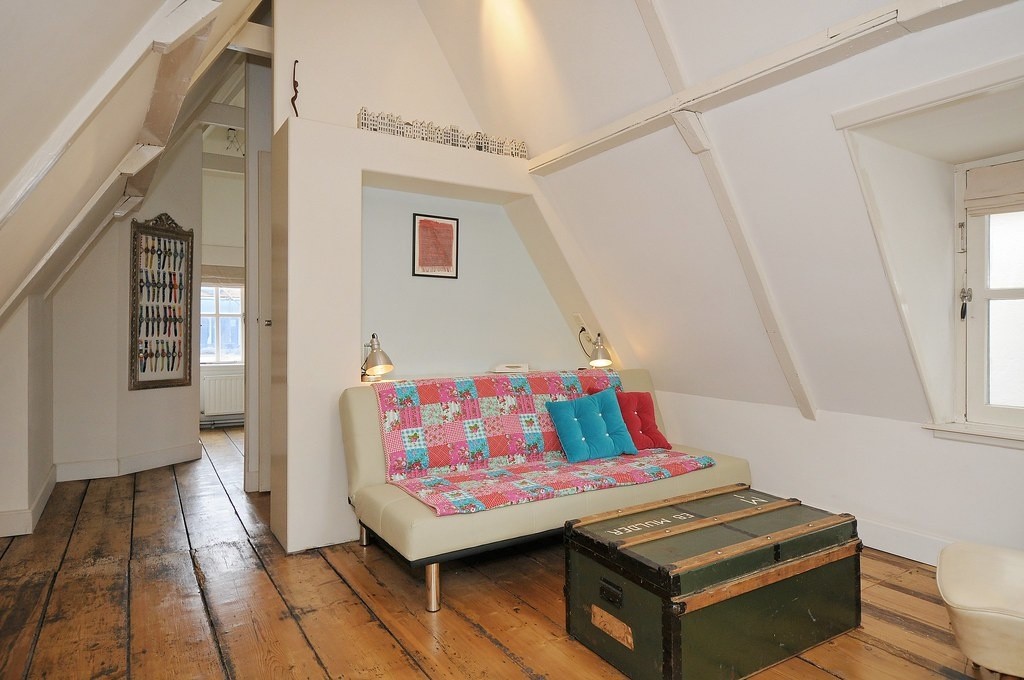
[337,368,752,612]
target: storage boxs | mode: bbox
[560,482,861,680]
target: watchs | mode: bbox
[140,235,184,271]
[139,268,184,304]
[139,340,182,372]
[139,305,183,337]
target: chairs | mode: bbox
[936,541,1024,680]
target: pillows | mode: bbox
[545,386,638,462]
[617,391,671,450]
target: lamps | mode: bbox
[361,333,394,382]
[588,332,612,368]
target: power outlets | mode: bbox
[574,313,593,343]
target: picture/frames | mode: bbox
[412,212,459,279]
[128,212,194,390]
